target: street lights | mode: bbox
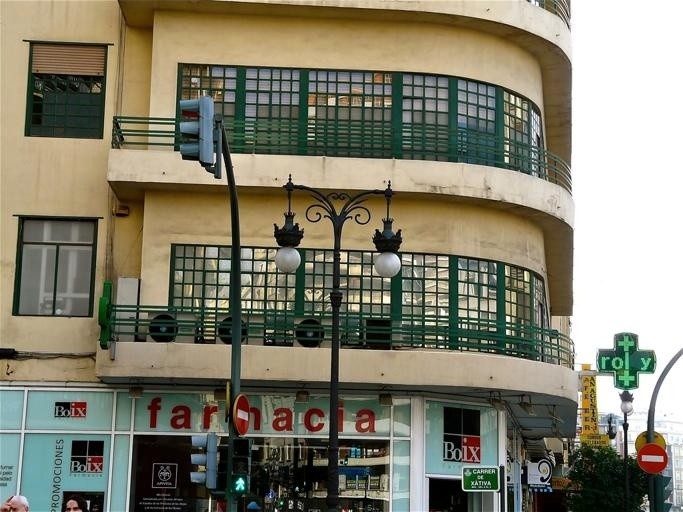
[607,413,618,448]
[618,388,637,511]
[268,448,281,492]
[271,174,402,512]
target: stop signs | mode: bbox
[637,443,669,474]
[233,394,252,435]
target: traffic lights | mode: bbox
[178,95,211,166]
[229,438,252,496]
[344,454,349,465]
[189,431,215,488]
[277,496,285,507]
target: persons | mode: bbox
[62,494,86,512]
[0,496,29,511]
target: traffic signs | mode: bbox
[461,466,500,493]
